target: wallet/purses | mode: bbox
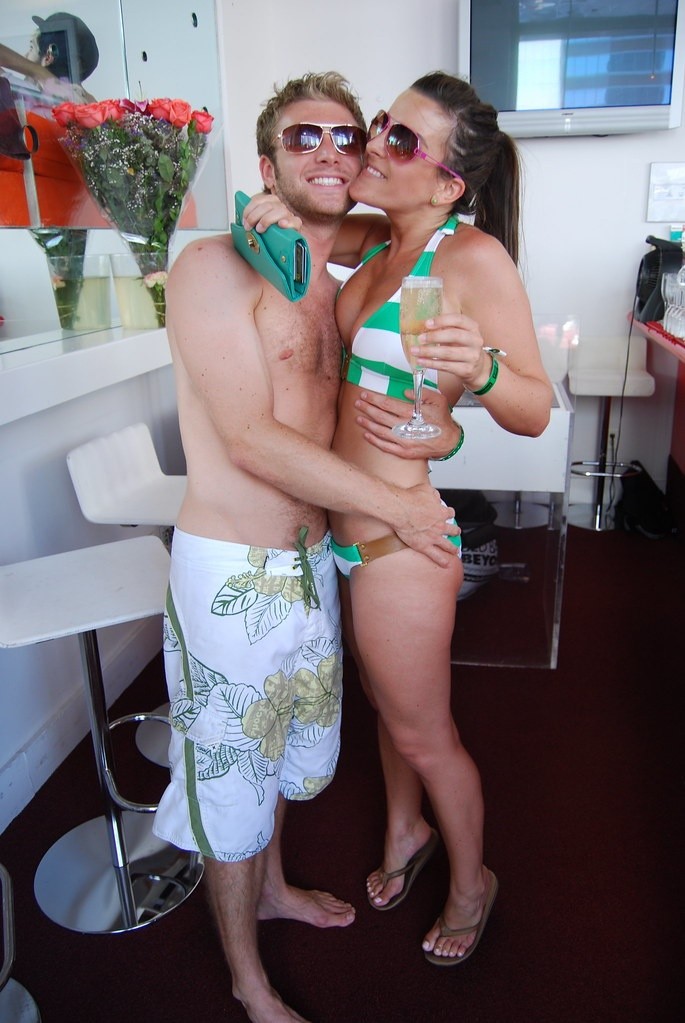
[230,191,312,303]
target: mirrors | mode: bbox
[0,0,229,231]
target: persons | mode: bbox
[152,75,465,1023]
[24,12,99,104]
[242,70,553,966]
[0,44,88,103]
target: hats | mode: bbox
[33,12,99,82]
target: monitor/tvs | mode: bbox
[458,0,685,137]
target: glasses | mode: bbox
[267,122,367,156]
[366,109,462,180]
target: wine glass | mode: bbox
[660,272,685,339]
[390,275,444,440]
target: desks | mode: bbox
[628,310,685,532]
[429,331,575,671]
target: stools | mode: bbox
[0,535,205,934]
[492,334,566,529]
[65,422,187,768]
[567,333,655,532]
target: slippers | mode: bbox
[424,870,499,966]
[367,828,438,910]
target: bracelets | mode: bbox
[462,347,506,396]
[430,423,464,461]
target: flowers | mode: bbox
[52,97,216,328]
